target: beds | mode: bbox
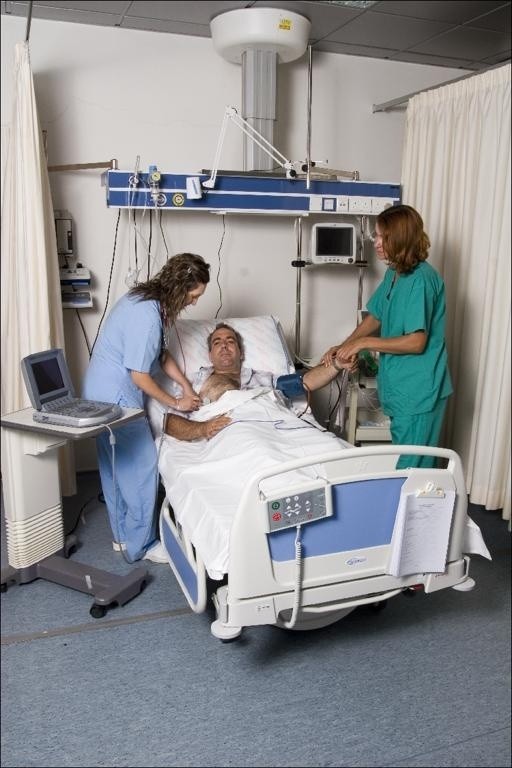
[141,316,471,644]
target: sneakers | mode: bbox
[140,542,170,563]
[112,540,127,552]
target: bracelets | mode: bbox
[333,358,343,372]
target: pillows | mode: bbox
[150,317,289,376]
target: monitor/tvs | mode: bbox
[20,348,78,410]
[311,222,357,265]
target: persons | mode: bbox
[162,322,361,441]
[81,253,211,564]
[319,205,454,469]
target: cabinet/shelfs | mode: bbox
[335,359,392,446]
